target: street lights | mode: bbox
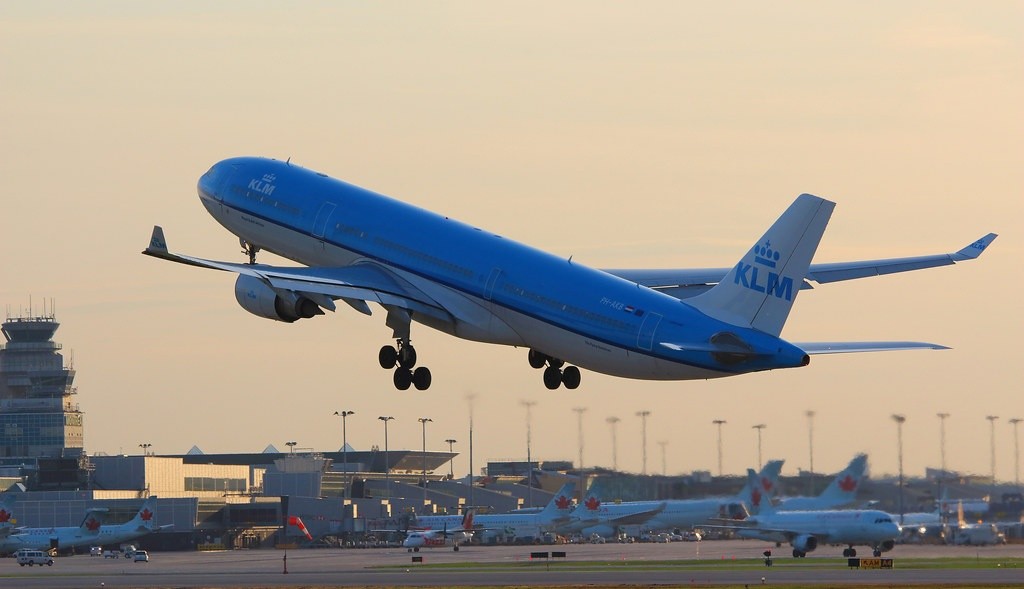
[891,414,906,490]
[285,441,297,457]
[378,416,395,500]
[937,413,950,469]
[139,444,152,455]
[1010,418,1024,486]
[637,411,650,475]
[986,416,999,481]
[752,424,765,472]
[445,439,457,474]
[418,418,433,500]
[333,409,355,485]
[713,420,727,477]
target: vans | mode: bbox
[13,549,53,566]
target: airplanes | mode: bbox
[403,455,1024,552]
[0,495,160,557]
[141,156,999,390]
[738,468,901,557]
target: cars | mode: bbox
[104,543,149,563]
[90,546,101,556]
